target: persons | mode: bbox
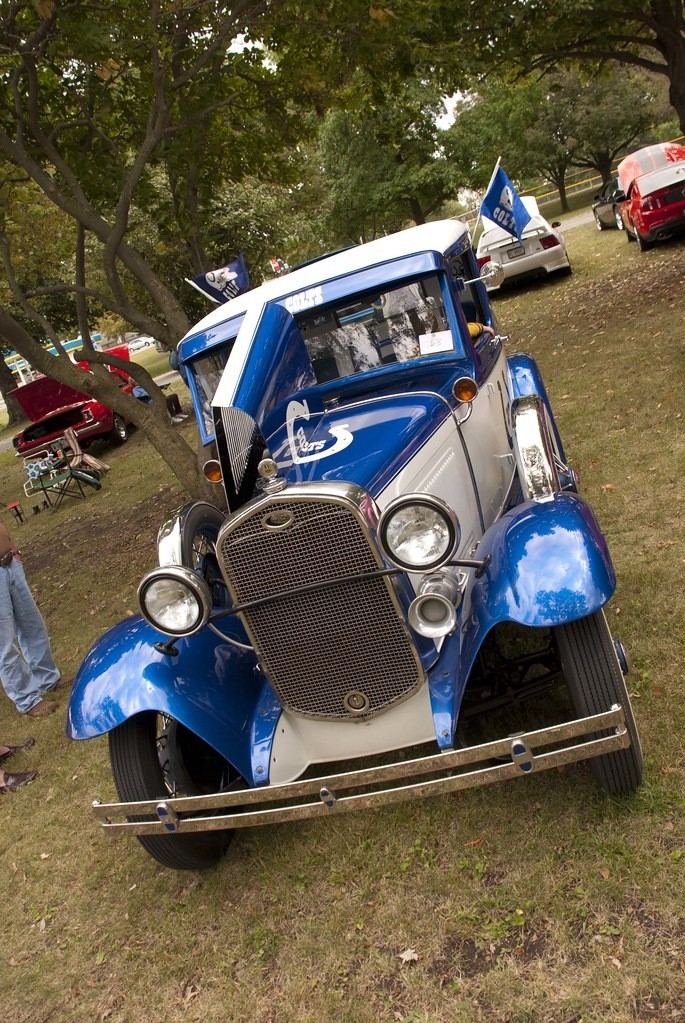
[0,518,75,717]
[467,322,494,337]
[0,738,38,794]
[130,376,188,423]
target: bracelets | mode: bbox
[12,551,21,555]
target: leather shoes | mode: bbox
[0,771,40,795]
[0,738,34,767]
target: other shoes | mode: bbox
[53,677,74,692]
[24,700,59,719]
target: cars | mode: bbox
[591,177,626,231]
[615,142,685,251]
[64,218,645,867]
[7,343,141,461]
[475,196,573,299]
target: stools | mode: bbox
[6,501,27,525]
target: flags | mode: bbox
[191,256,249,303]
[480,164,532,253]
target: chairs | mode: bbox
[22,428,110,512]
